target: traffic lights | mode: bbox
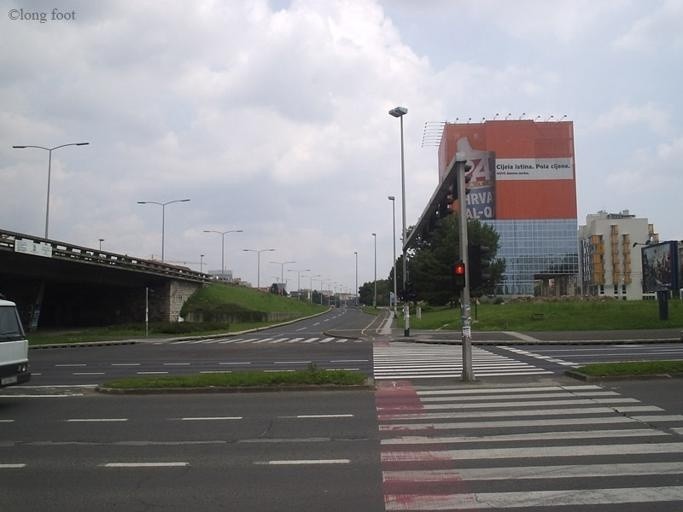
[428,164,473,232]
[454,262,465,289]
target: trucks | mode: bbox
[0,299,31,387]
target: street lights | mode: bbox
[372,233,377,308]
[388,107,410,336]
[204,230,243,280]
[354,252,358,306]
[200,255,204,272]
[99,239,104,251]
[243,249,274,290]
[270,261,352,306]
[13,143,89,239]
[137,199,191,262]
